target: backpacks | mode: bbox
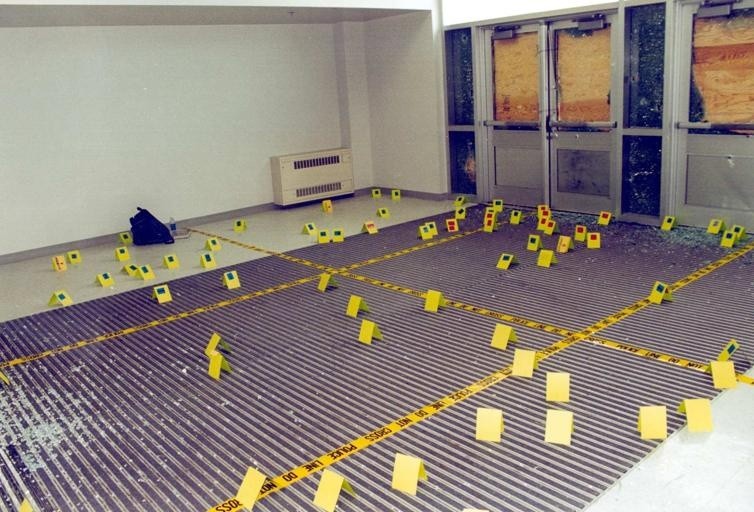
[130,207,174,245]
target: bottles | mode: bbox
[168,216,177,236]
